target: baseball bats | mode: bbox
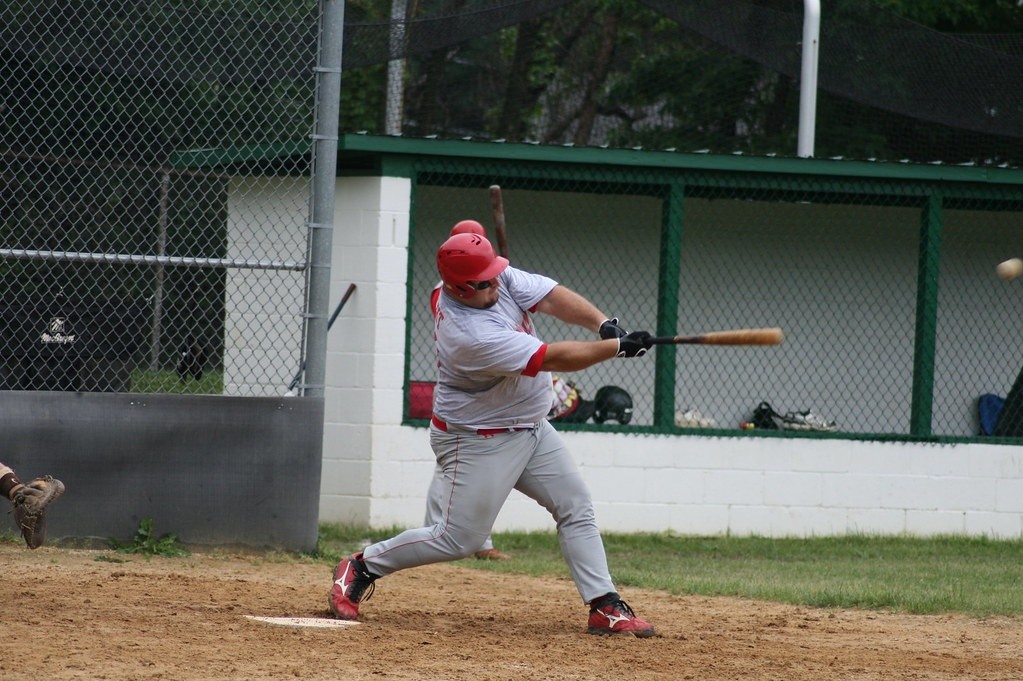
[644,326,783,346]
[487,185,511,267]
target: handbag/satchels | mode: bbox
[751,401,782,430]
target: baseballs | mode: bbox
[996,258,1023,280]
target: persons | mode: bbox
[0,463,64,549]
[328,227,656,637]
[425,221,514,559]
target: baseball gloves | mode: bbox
[10,475,66,549]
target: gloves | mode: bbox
[615,331,653,358]
[597,317,629,340]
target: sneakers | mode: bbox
[328,551,376,621]
[587,592,656,637]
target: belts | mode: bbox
[432,413,528,436]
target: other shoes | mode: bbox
[475,550,510,561]
[675,409,716,427]
[784,409,840,432]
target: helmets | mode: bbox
[594,386,633,424]
[436,232,509,298]
[449,219,487,238]
